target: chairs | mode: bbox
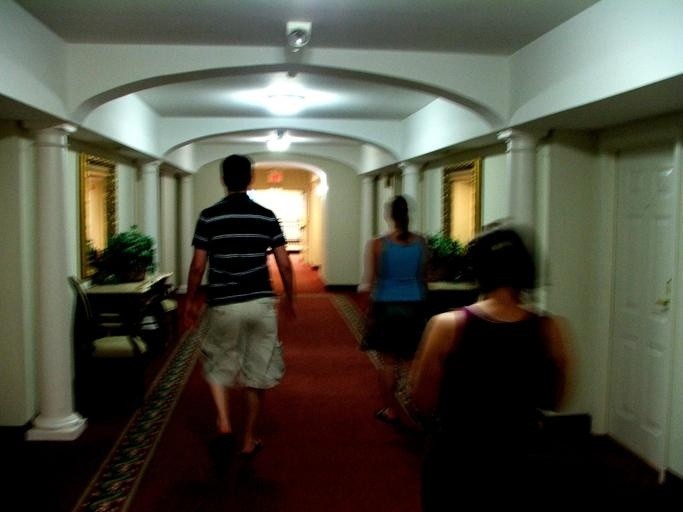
[69,277,148,415]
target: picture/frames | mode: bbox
[440,157,481,249]
[79,151,116,279]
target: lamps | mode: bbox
[285,20,313,56]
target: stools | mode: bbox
[156,298,179,341]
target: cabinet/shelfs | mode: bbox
[79,272,174,336]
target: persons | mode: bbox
[178,152,297,457]
[353,192,430,430]
[401,225,573,512]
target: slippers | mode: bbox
[240,438,264,457]
[376,408,398,424]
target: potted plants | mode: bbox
[421,231,465,282]
[98,226,155,282]
